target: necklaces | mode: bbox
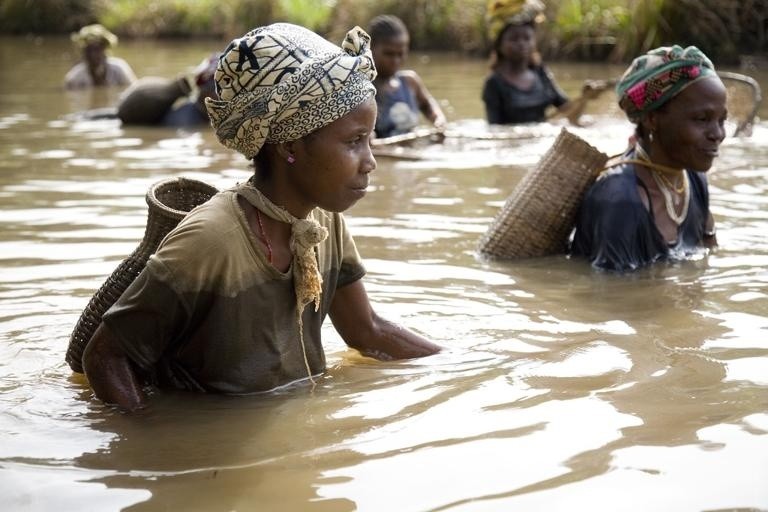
[254,209,274,267]
[633,144,693,224]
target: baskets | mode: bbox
[479,126,613,276]
[63,177,233,380]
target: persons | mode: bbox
[61,24,138,95]
[158,54,228,134]
[562,42,729,275]
[82,21,450,423]
[357,13,449,154]
[480,13,610,131]
